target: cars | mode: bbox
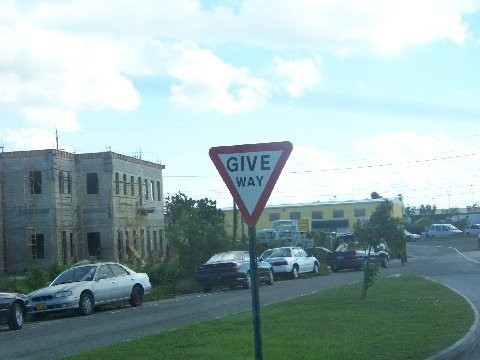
[193,248,275,293]
[0,289,29,331]
[259,246,320,279]
[23,261,152,317]
[324,241,390,273]
[465,224,480,236]
[403,228,421,241]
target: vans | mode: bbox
[428,223,463,237]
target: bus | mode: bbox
[257,226,316,248]
